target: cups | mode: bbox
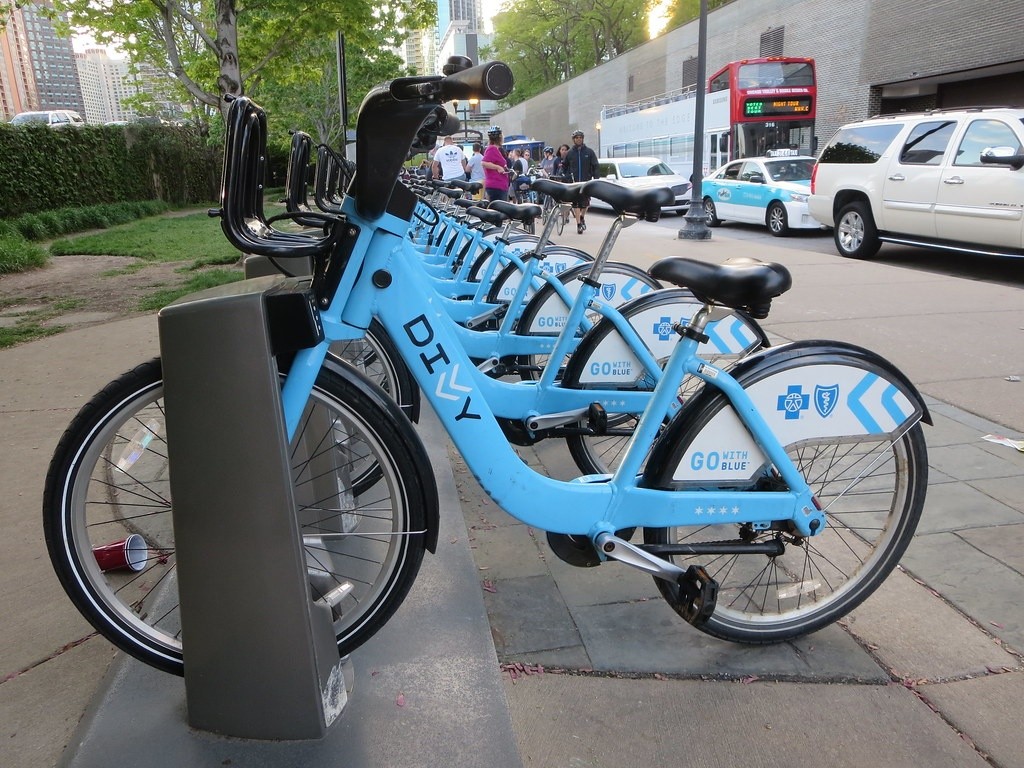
[93,534,148,572]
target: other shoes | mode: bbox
[577,223,583,233]
[580,217,586,230]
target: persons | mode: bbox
[481,125,509,202]
[432,137,467,187]
[504,148,539,204]
[553,144,571,225]
[563,130,599,234]
[423,144,483,199]
[465,142,485,201]
[540,146,558,179]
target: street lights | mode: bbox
[452,96,478,142]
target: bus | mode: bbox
[599,56,818,183]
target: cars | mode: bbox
[589,158,692,216]
[701,156,835,237]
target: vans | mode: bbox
[6,110,83,128]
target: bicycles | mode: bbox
[542,171,574,236]
[40,54,934,680]
[503,164,544,235]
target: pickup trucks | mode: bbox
[808,106,1024,258]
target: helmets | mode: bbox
[571,130,584,139]
[543,147,553,154]
[488,126,502,136]
